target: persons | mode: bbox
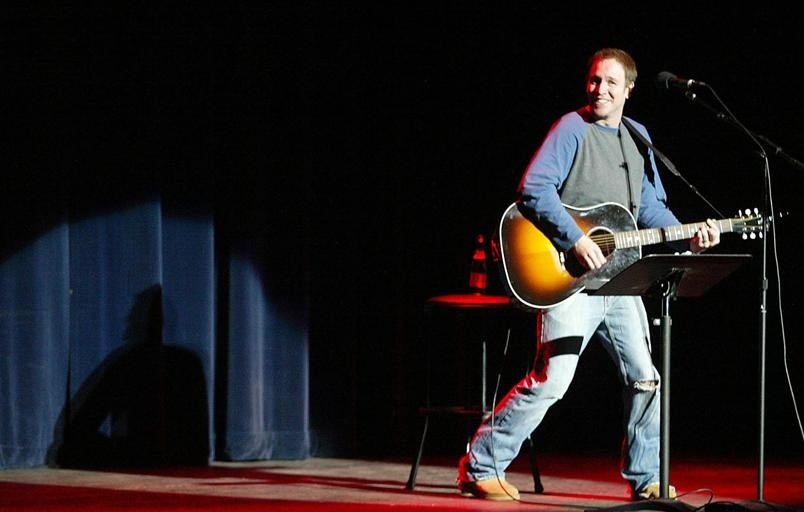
[456,47,721,505]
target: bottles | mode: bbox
[470,236,488,296]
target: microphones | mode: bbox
[656,71,706,88]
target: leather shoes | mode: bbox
[461,478,520,501]
[639,483,676,501]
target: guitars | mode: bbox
[497,194,774,310]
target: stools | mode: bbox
[404,290,544,494]
